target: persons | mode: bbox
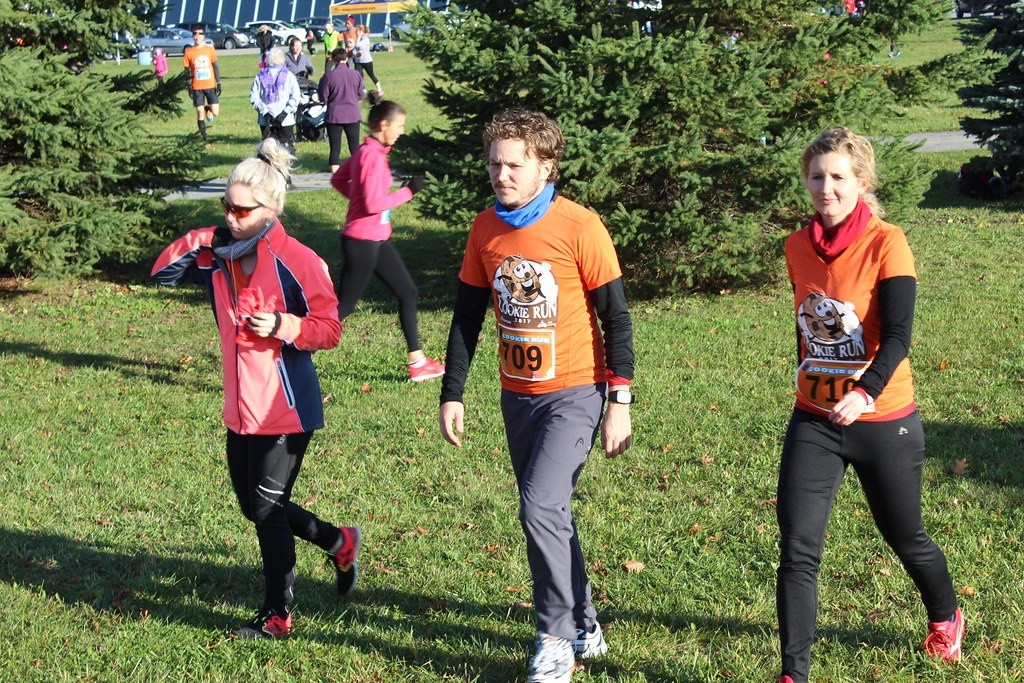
[331,89,446,381]
[778,125,966,683]
[151,135,362,636]
[151,16,384,191]
[440,109,635,683]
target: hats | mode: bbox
[346,38,354,44]
[346,17,354,25]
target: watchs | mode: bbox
[607,390,635,404]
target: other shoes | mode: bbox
[204,142,210,148]
[205,102,213,122]
[377,90,383,96]
[315,48,317,52]
[309,55,314,57]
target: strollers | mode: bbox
[294,75,328,141]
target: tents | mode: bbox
[329,0,419,53]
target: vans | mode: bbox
[174,22,250,49]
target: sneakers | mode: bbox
[525,630,575,683]
[228,612,291,640]
[779,675,794,683]
[572,620,608,659]
[922,608,965,662]
[330,525,360,594]
[408,357,446,382]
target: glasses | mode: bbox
[220,196,262,218]
[193,31,203,35]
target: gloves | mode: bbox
[272,110,287,127]
[263,112,275,124]
[188,85,194,99]
[306,66,313,75]
[407,175,426,195]
[216,84,222,97]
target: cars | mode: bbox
[295,17,346,41]
[237,28,259,47]
[102,31,137,60]
[383,20,431,41]
[954,0,1006,18]
[137,28,215,55]
[245,20,307,46]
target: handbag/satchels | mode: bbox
[265,119,296,156]
